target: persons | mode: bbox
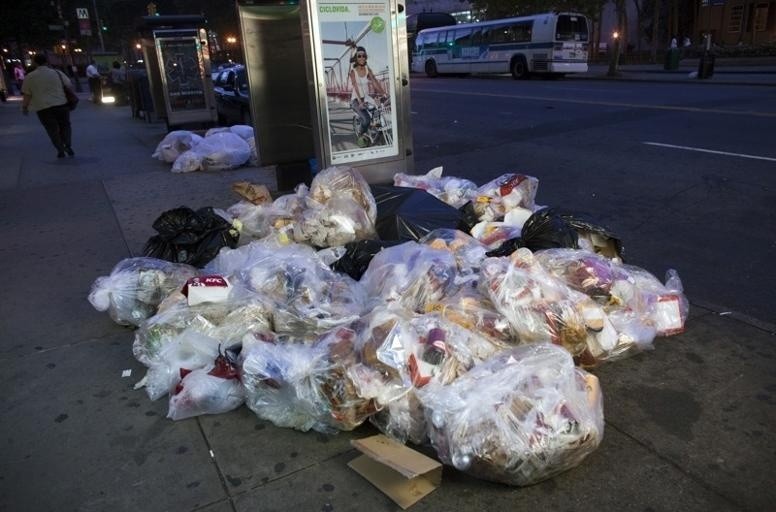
[669,34,692,48]
[349,46,388,148]
[108,61,127,107]
[85,59,102,102]
[15,61,59,96]
[21,53,75,158]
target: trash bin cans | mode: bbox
[698,49,716,78]
[664,47,680,70]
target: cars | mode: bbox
[212,61,251,125]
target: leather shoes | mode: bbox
[65,147,74,156]
[57,151,64,158]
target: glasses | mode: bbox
[358,55,365,58]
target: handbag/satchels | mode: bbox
[55,70,79,111]
[364,95,378,111]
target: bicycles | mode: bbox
[352,96,394,147]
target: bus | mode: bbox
[405,12,590,80]
